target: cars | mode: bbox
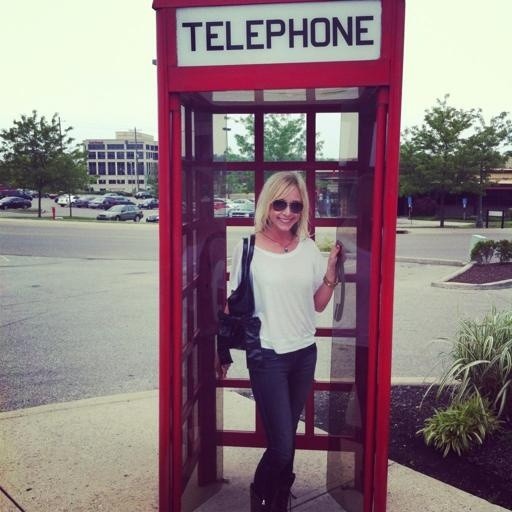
[181,195,256,218]
[44,191,159,221]
[0,188,39,210]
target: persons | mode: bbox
[217,171,341,512]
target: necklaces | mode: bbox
[259,231,293,252]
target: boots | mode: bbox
[248,475,295,512]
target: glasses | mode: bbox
[269,199,303,213]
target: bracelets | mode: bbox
[323,274,338,288]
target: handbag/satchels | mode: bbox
[229,233,256,317]
